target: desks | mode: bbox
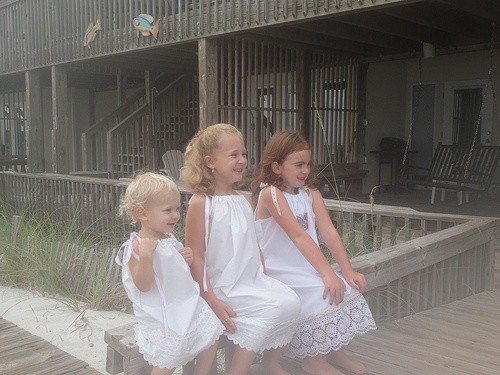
[377,150,417,195]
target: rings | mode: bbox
[225,317,230,321]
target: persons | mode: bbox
[252,131,378,375]
[116,171,226,375]
[179,124,302,375]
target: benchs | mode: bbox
[409,141,500,208]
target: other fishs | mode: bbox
[81,18,103,49]
[131,14,160,40]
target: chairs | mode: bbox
[162,149,187,183]
